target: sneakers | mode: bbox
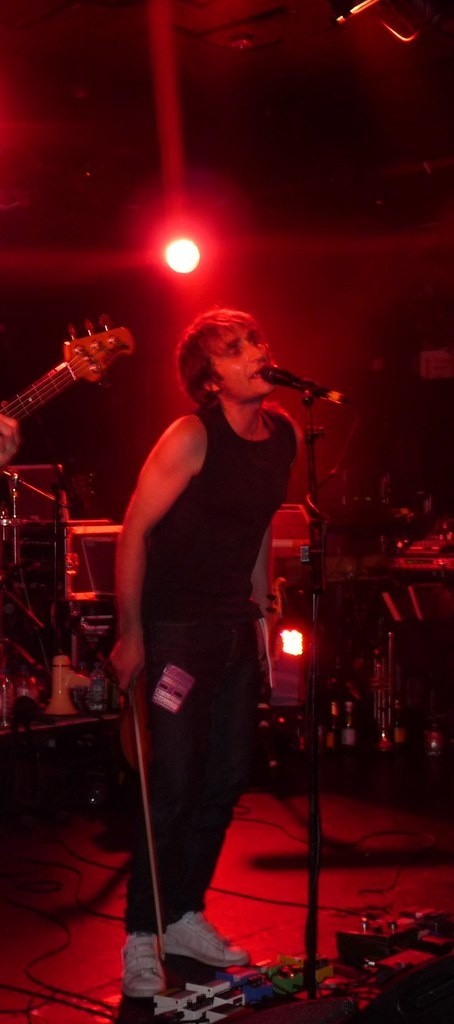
[121,930,162,998]
[162,910,250,967]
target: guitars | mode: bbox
[0,313,136,441]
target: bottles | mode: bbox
[0,664,41,729]
[72,660,122,713]
[252,693,454,772]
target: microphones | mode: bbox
[260,365,350,405]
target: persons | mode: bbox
[104,307,304,998]
[0,413,22,466]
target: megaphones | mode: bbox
[43,655,103,716]
